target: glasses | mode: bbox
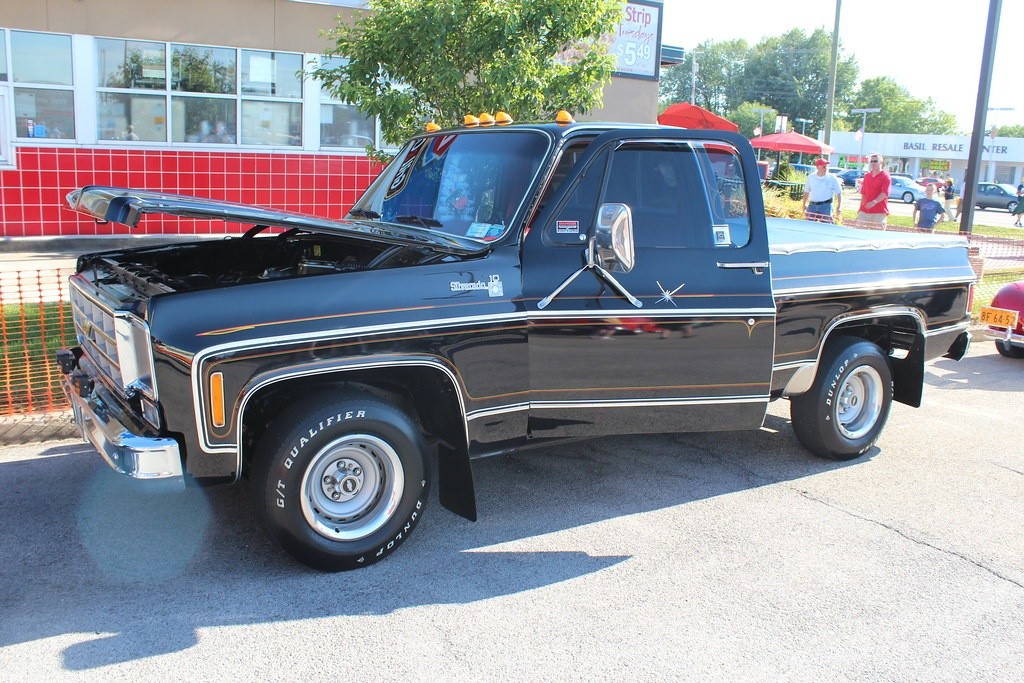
[871,160,878,163]
[823,166,827,168]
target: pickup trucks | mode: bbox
[53,111,976,574]
[836,168,866,186]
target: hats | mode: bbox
[816,158,830,166]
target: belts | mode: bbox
[812,200,830,205]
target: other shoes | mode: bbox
[1014,222,1018,227]
[1019,223,1024,227]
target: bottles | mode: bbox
[27,120,34,138]
[126,125,136,141]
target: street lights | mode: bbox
[986,107,1016,183]
[752,109,775,160]
[851,108,880,169]
[794,118,814,164]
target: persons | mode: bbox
[913,183,945,234]
[990,179,997,190]
[933,172,949,180]
[802,158,842,225]
[955,175,967,221]
[854,155,891,230]
[939,178,957,222]
[1012,177,1024,227]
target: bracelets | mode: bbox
[872,199,877,204]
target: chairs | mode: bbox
[627,167,690,246]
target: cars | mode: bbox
[890,173,914,180]
[915,176,948,191]
[757,161,843,181]
[858,176,927,203]
[979,281,1024,358]
[974,182,1020,212]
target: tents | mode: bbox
[750,126,833,162]
[658,102,740,162]
